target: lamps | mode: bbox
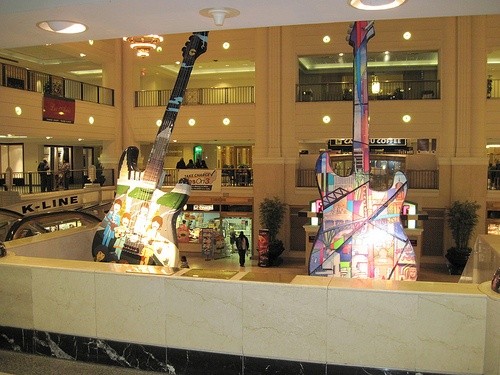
[122,35,163,57]
[199,7,240,26]
[370,75,381,93]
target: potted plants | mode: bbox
[257,199,287,266]
[446,200,479,275]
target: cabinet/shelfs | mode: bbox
[202,226,228,261]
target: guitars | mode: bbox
[92,31,209,267]
[308,21,418,281]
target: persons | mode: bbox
[394,88,403,99]
[229,165,251,186]
[176,158,208,169]
[236,231,249,266]
[180,256,189,268]
[39,160,53,192]
[55,170,60,189]
[62,160,70,190]
[488,163,500,190]
